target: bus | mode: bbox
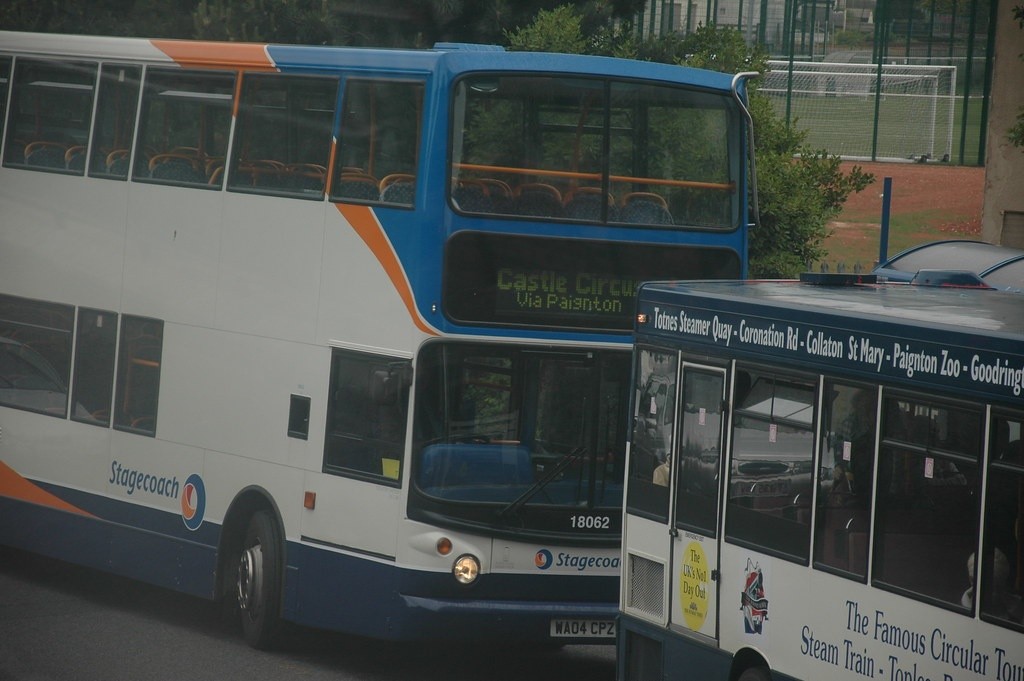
[609,268,1024,681]
[0,29,761,651]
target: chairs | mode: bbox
[5,136,676,224]
[728,474,993,611]
